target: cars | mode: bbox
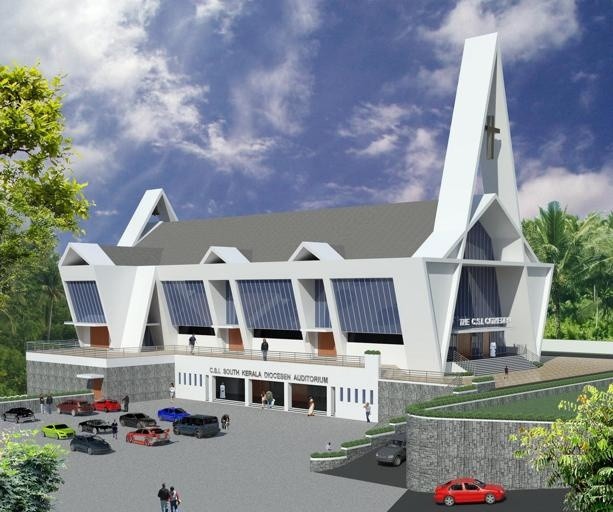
[91,399,122,414]
[67,433,112,456]
[374,439,405,467]
[2,407,36,425]
[76,418,112,435]
[55,400,93,417]
[40,422,75,441]
[432,476,505,507]
[157,405,189,423]
[118,412,156,429]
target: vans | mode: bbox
[172,413,222,441]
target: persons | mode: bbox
[326,442,332,451]
[122,395,129,412]
[307,396,315,416]
[169,486,179,512]
[46,395,52,414]
[112,419,118,439]
[261,338,268,361]
[364,402,371,422]
[40,394,45,414]
[504,366,508,379]
[168,383,175,401]
[158,484,170,512]
[189,333,197,354]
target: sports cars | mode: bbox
[124,427,172,447]
